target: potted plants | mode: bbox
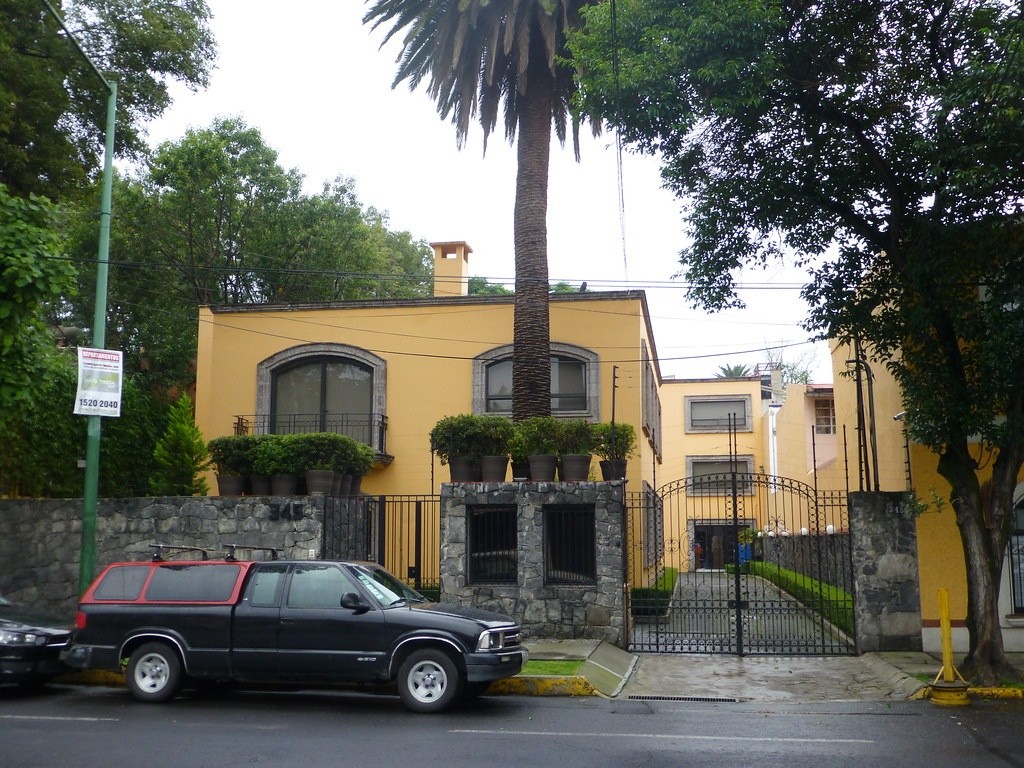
[427,413,636,482]
[205,432,374,498]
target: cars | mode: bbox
[0,596,72,688]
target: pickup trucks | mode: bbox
[69,544,528,714]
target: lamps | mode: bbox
[768,531,776,540]
[758,531,763,539]
[782,531,789,540]
[800,528,808,540]
[826,525,836,543]
